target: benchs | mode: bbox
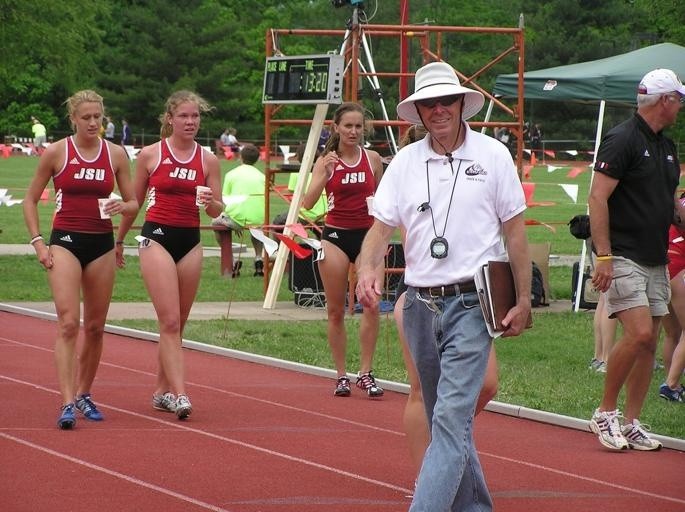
[106,224,325,281]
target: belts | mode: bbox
[418,279,477,299]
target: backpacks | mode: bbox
[530,261,549,307]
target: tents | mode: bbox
[476,43,685,312]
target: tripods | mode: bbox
[339,9,401,157]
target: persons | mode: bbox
[114,90,225,419]
[305,103,385,396]
[100,116,134,146]
[273,125,332,261]
[587,192,685,403]
[354,60,534,512]
[31,115,46,154]
[24,88,138,429]
[587,68,685,450]
[220,128,243,159]
[211,145,271,278]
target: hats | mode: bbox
[396,62,485,126]
[639,69,685,95]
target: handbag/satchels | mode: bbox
[572,271,600,309]
[567,215,590,239]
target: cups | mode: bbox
[196,185,211,207]
[366,196,374,216]
[98,198,112,219]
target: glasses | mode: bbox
[415,94,461,107]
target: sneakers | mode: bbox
[334,375,351,395]
[590,359,608,372]
[152,390,176,412]
[589,407,629,450]
[232,259,242,278]
[74,393,103,420]
[620,419,663,451]
[175,394,192,419]
[659,384,685,401]
[57,403,76,429]
[254,256,264,277]
[356,370,384,396]
